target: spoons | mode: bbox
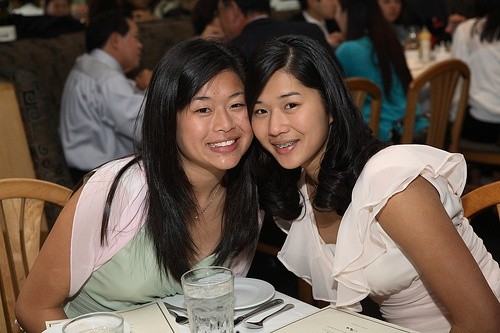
[168,310,189,325]
[246,304,295,329]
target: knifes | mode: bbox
[234,299,284,324]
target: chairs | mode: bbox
[346,59,500,223]
[0,177,73,333]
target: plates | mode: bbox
[407,64,423,69]
[42,320,130,333]
[234,277,275,310]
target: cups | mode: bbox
[62,311,124,333]
[181,266,235,333]
[405,50,419,66]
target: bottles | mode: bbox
[406,27,431,63]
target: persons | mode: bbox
[15,36,265,333]
[0,0,500,218]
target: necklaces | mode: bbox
[190,185,222,219]
[244,34,500,333]
